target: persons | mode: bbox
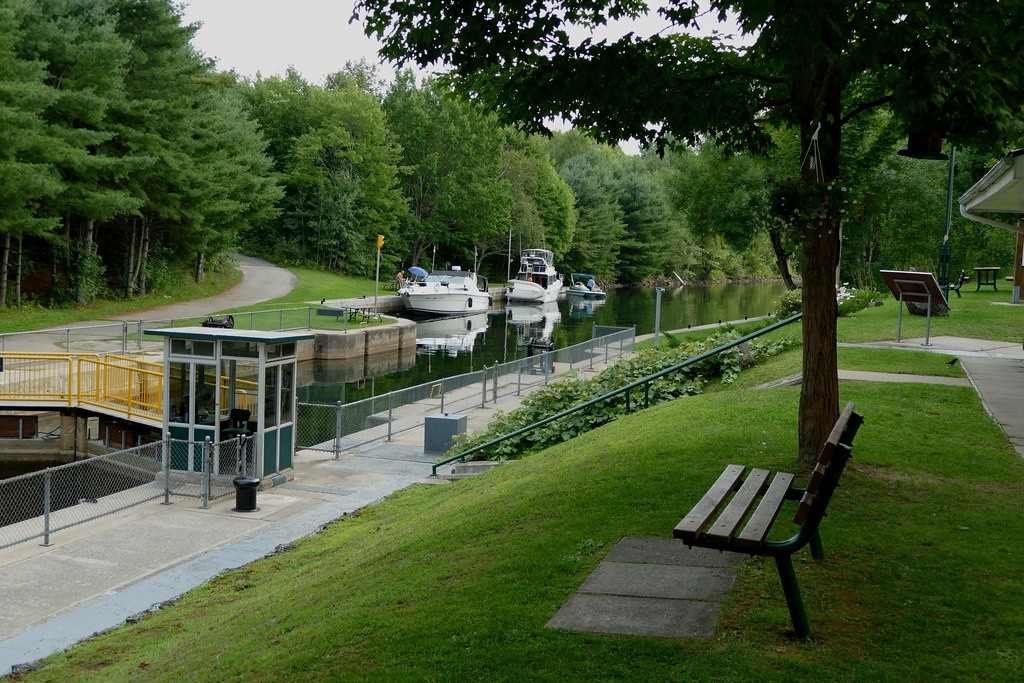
[574,278,595,290]
[395,270,414,286]
[527,273,532,281]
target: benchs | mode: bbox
[949,271,966,298]
[359,313,384,323]
[672,403,863,640]
[382,283,395,291]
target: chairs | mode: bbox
[222,409,250,439]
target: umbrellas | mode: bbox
[408,266,428,282]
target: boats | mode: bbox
[565,273,606,298]
[503,301,562,363]
[398,270,493,314]
[505,230,565,303]
[416,312,489,373]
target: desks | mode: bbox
[342,306,378,323]
[388,280,400,290]
[973,267,999,292]
